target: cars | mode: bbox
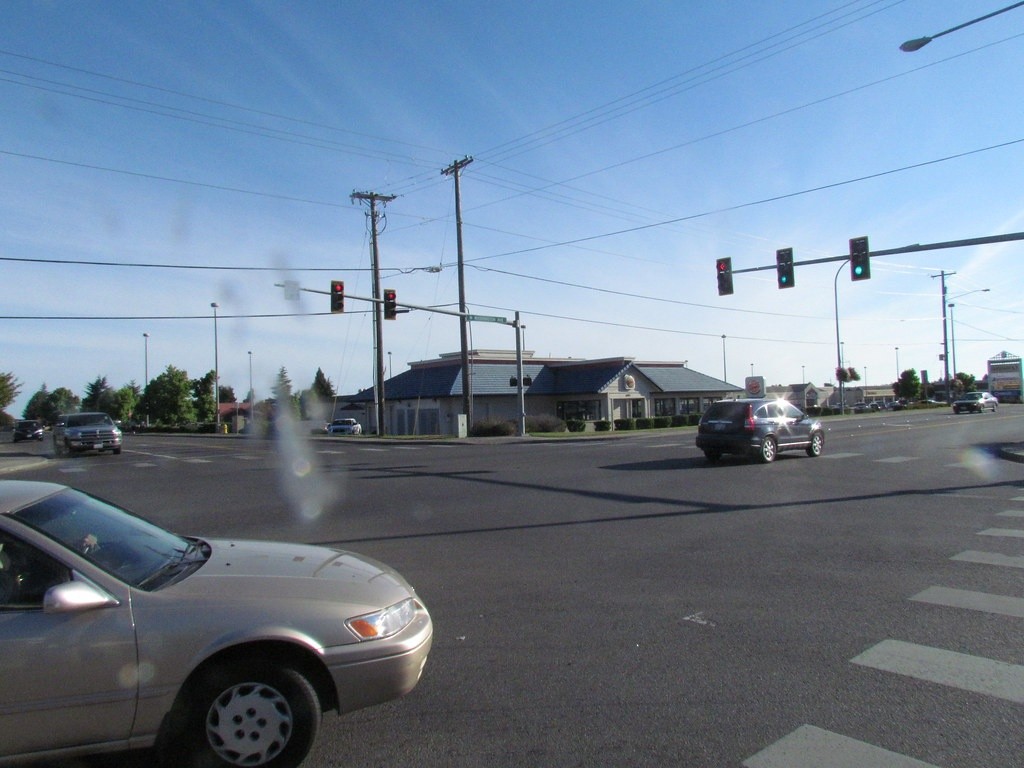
[833,399,942,410]
[952,391,999,413]
[0,478,433,768]
[13,421,45,443]
[327,418,361,434]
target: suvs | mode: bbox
[695,399,824,463]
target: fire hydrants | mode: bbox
[223,424,228,434]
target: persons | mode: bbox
[27,531,97,588]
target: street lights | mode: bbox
[144,333,153,421]
[802,366,804,384]
[948,303,956,381]
[751,364,753,375]
[834,242,920,412]
[864,367,867,400]
[941,288,990,406]
[388,351,392,378]
[895,347,899,382]
[248,350,255,421]
[840,341,844,414]
[722,335,728,383]
[212,302,224,431]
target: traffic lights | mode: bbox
[850,236,871,280]
[384,289,398,321]
[716,257,733,295]
[777,247,795,289]
[331,280,344,313]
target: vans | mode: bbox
[52,412,123,454]
